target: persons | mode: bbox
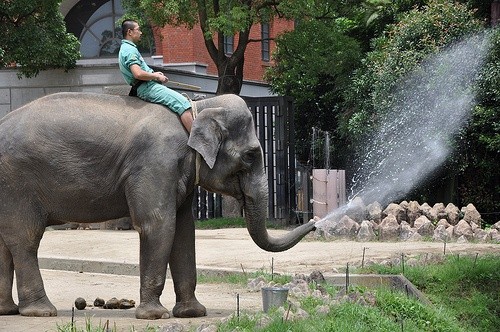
[119,19,194,135]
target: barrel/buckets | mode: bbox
[261,287,289,314]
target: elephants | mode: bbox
[0,93,316,320]
